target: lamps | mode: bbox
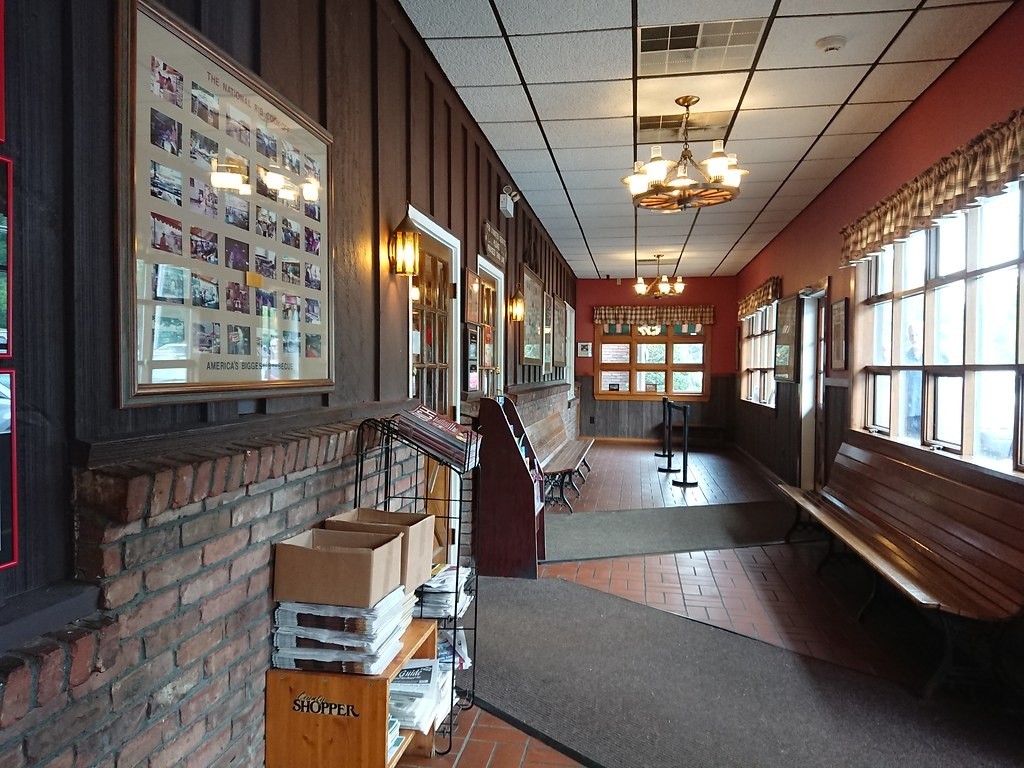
[621,96,749,214]
[634,254,686,299]
[509,283,525,322]
[388,201,422,277]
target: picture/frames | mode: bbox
[578,342,592,357]
[646,384,658,393]
[460,266,482,393]
[773,293,802,384]
[609,384,619,391]
[829,297,850,371]
[519,263,568,375]
[112,0,336,410]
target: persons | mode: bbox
[906,322,924,439]
[151,56,322,356]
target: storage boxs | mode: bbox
[324,507,435,595]
[273,529,404,609]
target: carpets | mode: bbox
[442,575,1024,768]
[538,499,834,565]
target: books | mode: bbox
[495,396,530,471]
[388,713,406,762]
[398,404,483,472]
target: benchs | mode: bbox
[665,420,729,450]
[777,428,1024,704]
[524,411,595,515]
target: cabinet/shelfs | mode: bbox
[478,395,546,580]
[354,413,482,756]
[264,618,438,768]
